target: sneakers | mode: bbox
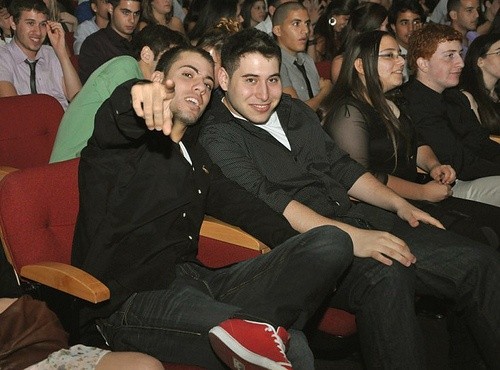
[209,315,299,370]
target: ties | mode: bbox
[24,59,39,95]
[295,60,321,123]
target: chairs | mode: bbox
[0,93,271,303]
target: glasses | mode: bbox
[377,53,405,60]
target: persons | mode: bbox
[1,0,500,56]
[324,30,500,253]
[399,24,500,207]
[271,2,334,128]
[0,290,166,370]
[50,23,190,164]
[457,32,500,142]
[72,45,354,370]
[0,0,82,114]
[202,27,499,370]
